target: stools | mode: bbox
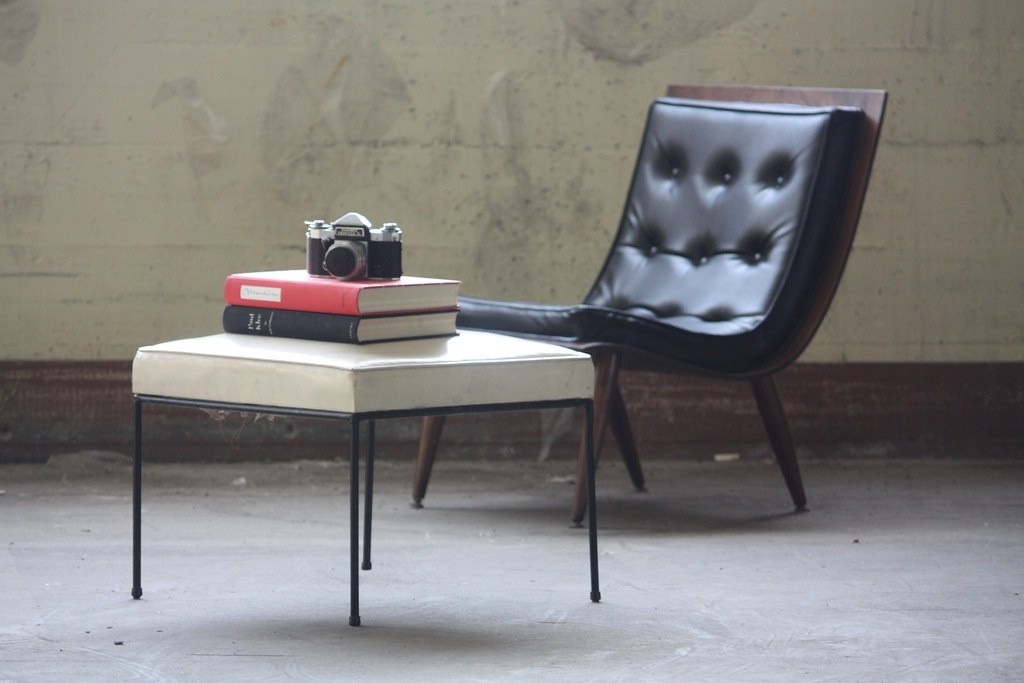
[128,333,602,627]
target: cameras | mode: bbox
[306,212,402,279]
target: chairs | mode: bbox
[410,83,890,527]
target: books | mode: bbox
[221,270,462,344]
